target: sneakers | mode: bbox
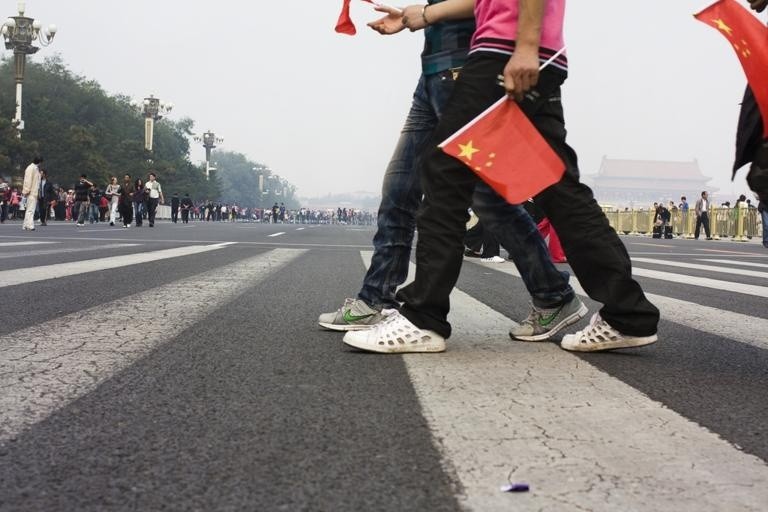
[561,311,658,352]
[509,299,589,342]
[342,311,446,353]
[318,298,395,331]
[481,256,504,263]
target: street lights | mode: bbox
[194,128,224,181]
[0,3,58,187]
[252,166,272,200]
[132,93,172,167]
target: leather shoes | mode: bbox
[464,249,480,257]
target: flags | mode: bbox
[690,0,768,139]
[438,94,566,207]
[332,1,371,36]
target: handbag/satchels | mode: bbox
[104,194,112,201]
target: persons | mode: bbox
[727,0,768,203]
[285,208,378,226]
[318,0,593,343]
[343,0,663,356]
[456,200,570,265]
[171,193,285,224]
[652,192,767,248]
[1,157,163,231]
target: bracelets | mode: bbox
[422,4,431,28]
